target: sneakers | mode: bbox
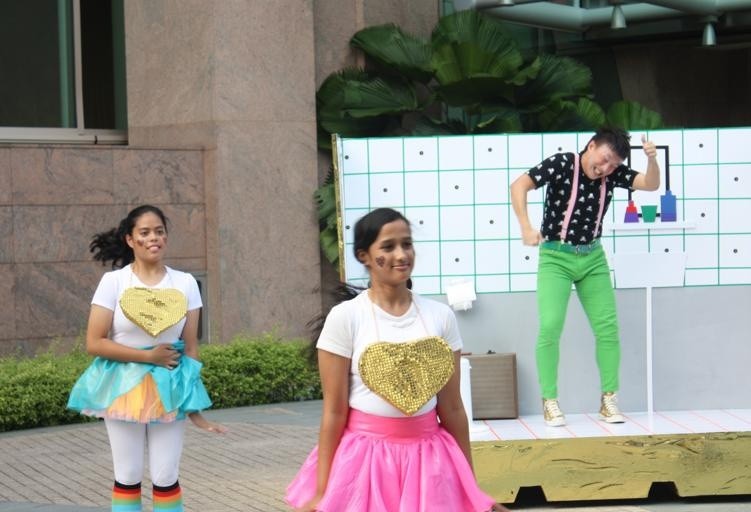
[597,390,626,424]
[542,394,567,427]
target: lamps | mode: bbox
[702,16,718,48]
[609,0,628,30]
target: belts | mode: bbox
[539,238,601,254]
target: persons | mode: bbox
[282,207,513,511]
[509,125,663,428]
[62,202,230,511]
